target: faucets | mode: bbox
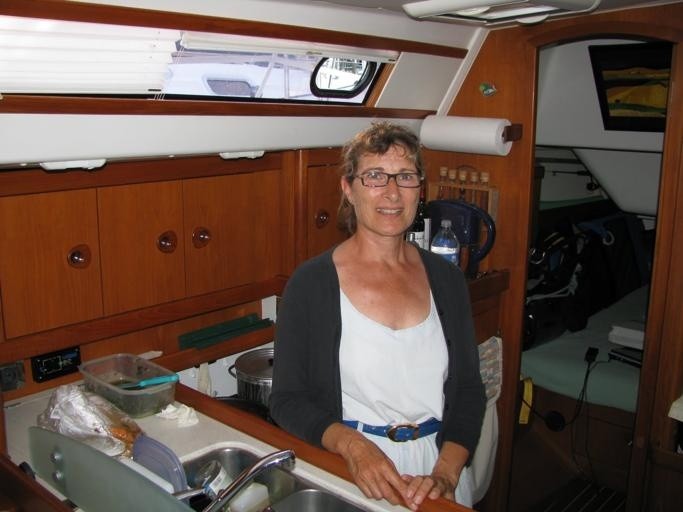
[202,447,296,512]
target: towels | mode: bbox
[471,337,504,407]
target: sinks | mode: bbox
[183,447,298,501]
[270,488,368,512]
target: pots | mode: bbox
[228,346,274,407]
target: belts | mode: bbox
[341,416,442,443]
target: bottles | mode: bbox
[194,460,232,500]
[402,179,430,253]
[430,218,461,267]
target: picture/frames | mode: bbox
[587,42,673,131]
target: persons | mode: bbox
[271,121,488,511]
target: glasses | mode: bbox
[349,169,425,188]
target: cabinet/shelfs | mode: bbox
[0,151,280,365]
[467,268,511,511]
[280,146,355,301]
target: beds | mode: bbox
[520,284,652,493]
